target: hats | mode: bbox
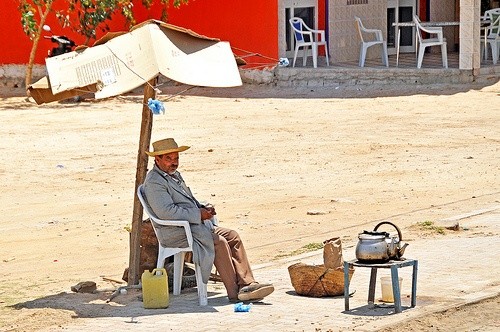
[145,138,191,157]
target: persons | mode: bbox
[141,138,274,304]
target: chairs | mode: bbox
[480,8,500,65]
[353,16,389,68]
[137,184,207,308]
[413,14,448,69]
[288,17,330,69]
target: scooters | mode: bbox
[42,25,81,102]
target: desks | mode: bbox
[343,259,418,314]
[392,19,491,67]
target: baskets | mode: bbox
[288,262,355,296]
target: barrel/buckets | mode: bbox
[381,276,403,302]
[141,268,169,309]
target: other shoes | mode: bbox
[230,297,263,303]
[238,283,274,300]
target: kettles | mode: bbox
[355,231,398,264]
[371,221,409,260]
